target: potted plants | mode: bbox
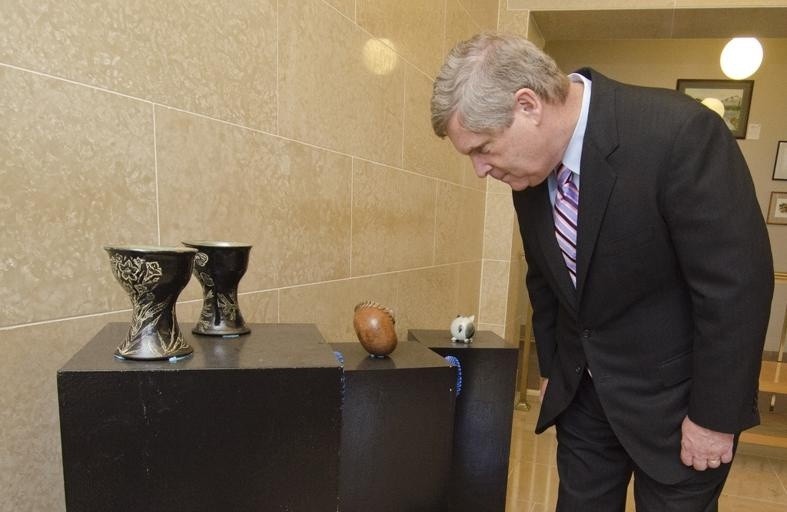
[674,77,754,141]
[764,139,787,226]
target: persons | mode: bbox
[429,30,778,511]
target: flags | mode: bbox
[102,237,256,365]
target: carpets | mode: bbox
[550,163,581,291]
